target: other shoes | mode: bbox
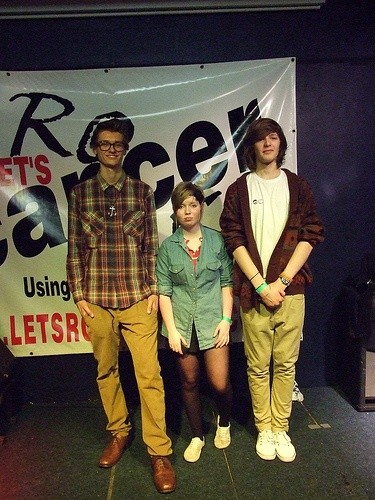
[291,381,304,402]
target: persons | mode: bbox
[68,119,176,492]
[156,183,235,463]
[292,318,325,401]
[220,118,325,462]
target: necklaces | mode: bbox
[106,190,119,211]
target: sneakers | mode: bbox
[256,429,276,460]
[184,436,205,462]
[214,415,231,449]
[272,430,296,462]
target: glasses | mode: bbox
[96,141,125,152]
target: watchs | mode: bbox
[279,273,291,286]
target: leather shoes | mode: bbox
[150,456,176,493]
[98,434,132,468]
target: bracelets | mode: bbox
[250,272,260,281]
[222,317,232,323]
[256,282,268,294]
